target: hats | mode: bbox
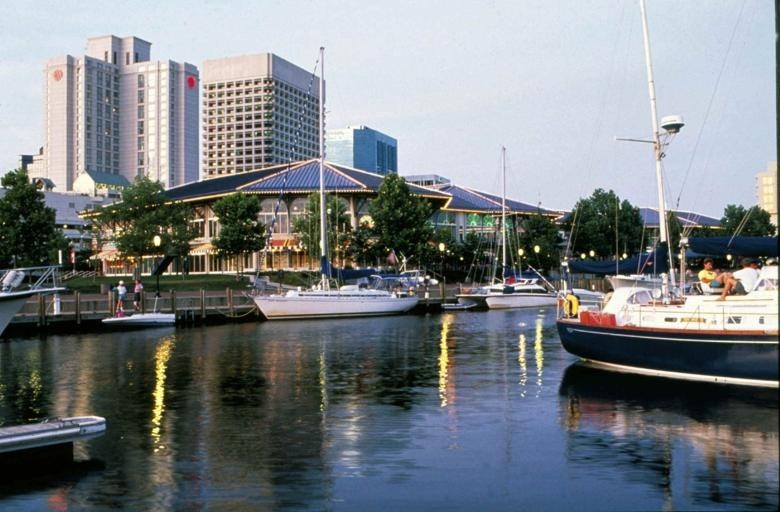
[751,260,762,268]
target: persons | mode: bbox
[391,285,415,299]
[115,280,127,312]
[133,279,143,311]
[697,257,762,301]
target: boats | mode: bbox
[558,202,606,306]
[441,302,478,311]
[0,265,66,331]
[605,190,694,294]
[102,311,175,327]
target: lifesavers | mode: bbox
[563,295,579,316]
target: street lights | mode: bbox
[153,235,162,298]
[439,242,446,280]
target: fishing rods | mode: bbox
[528,264,567,302]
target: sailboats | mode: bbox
[249,50,420,318]
[557,1,779,392]
[457,146,559,308]
